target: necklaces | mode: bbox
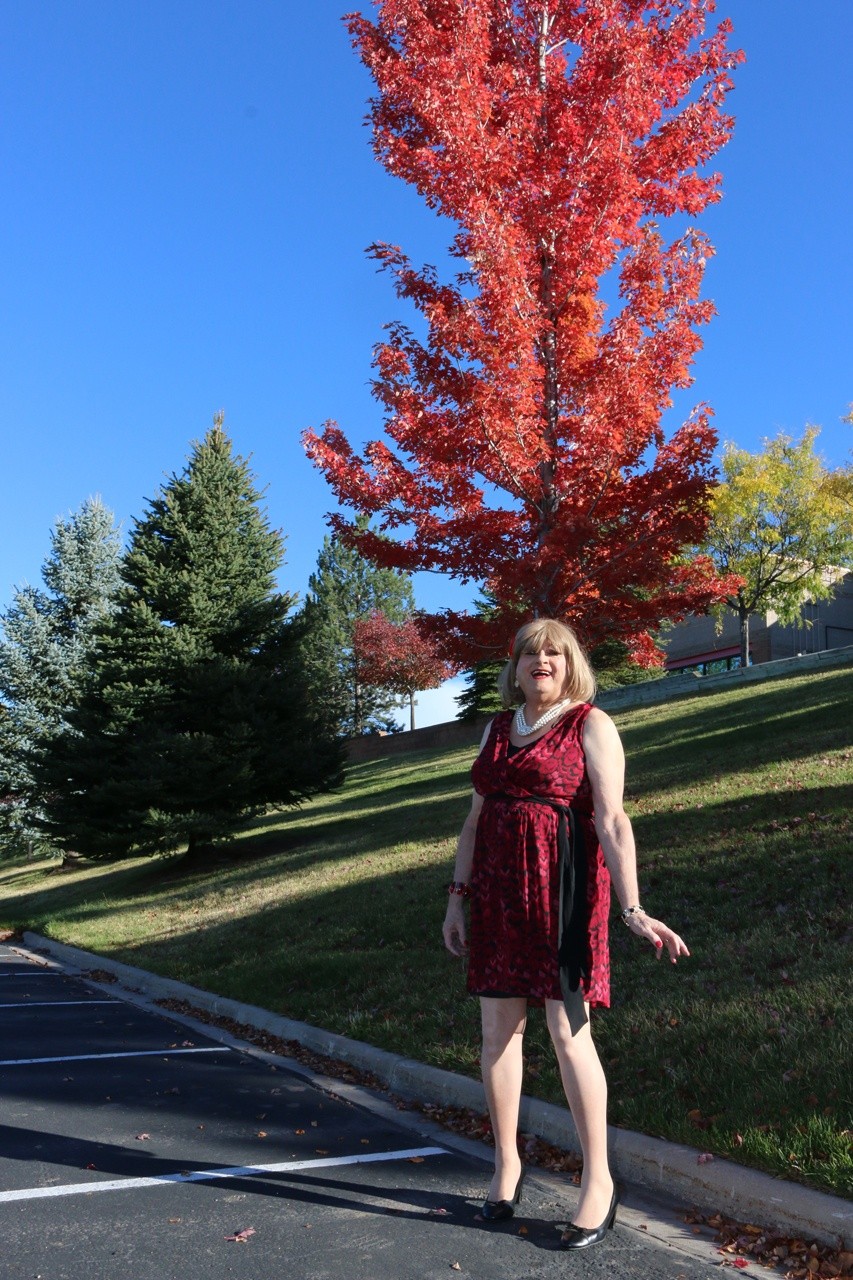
[516,697,572,737]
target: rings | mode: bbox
[664,939,669,943]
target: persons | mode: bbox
[440,618,690,1253]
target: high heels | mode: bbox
[482,1159,528,1223]
[561,1180,619,1250]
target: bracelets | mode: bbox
[621,905,646,928]
[447,880,472,898]
